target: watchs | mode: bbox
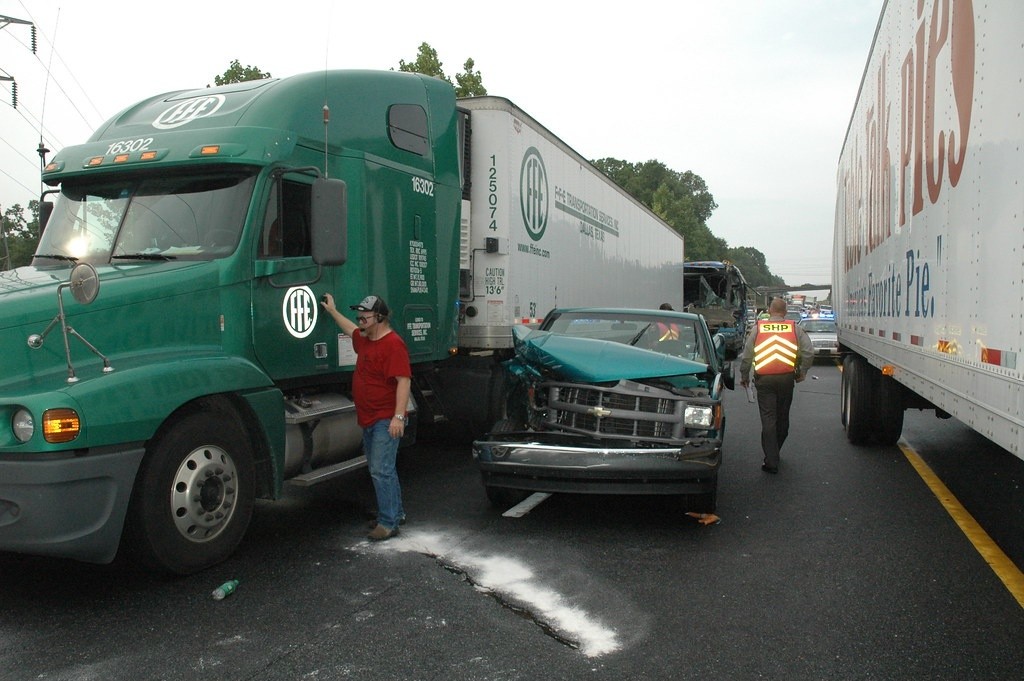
[395,414,405,421]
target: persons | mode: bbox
[655,303,681,340]
[740,298,814,473]
[321,292,412,542]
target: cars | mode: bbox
[748,293,841,367]
[471,306,727,523]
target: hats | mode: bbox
[350,295,388,315]
[660,303,673,310]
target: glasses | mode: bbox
[357,316,377,324]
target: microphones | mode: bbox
[360,321,379,332]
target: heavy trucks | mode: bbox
[1,68,683,580]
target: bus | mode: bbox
[682,260,761,359]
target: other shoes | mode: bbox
[367,513,406,528]
[761,463,778,474]
[367,523,397,538]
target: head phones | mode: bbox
[377,297,385,323]
